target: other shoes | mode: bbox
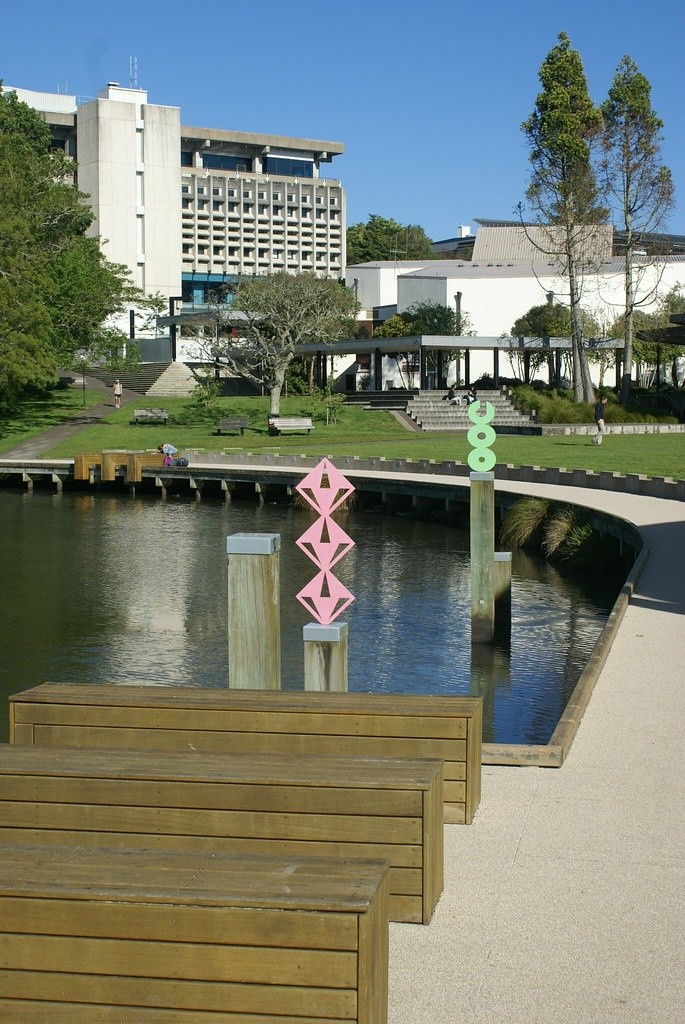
[591,440,595,445]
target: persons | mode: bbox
[157,443,177,466]
[113,380,122,408]
[448,385,461,405]
[593,395,608,445]
[467,386,477,405]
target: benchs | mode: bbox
[9,682,482,826]
[1,742,444,925]
[0,838,389,1023]
[268,417,314,437]
[71,377,88,386]
[133,409,168,424]
[213,418,250,437]
